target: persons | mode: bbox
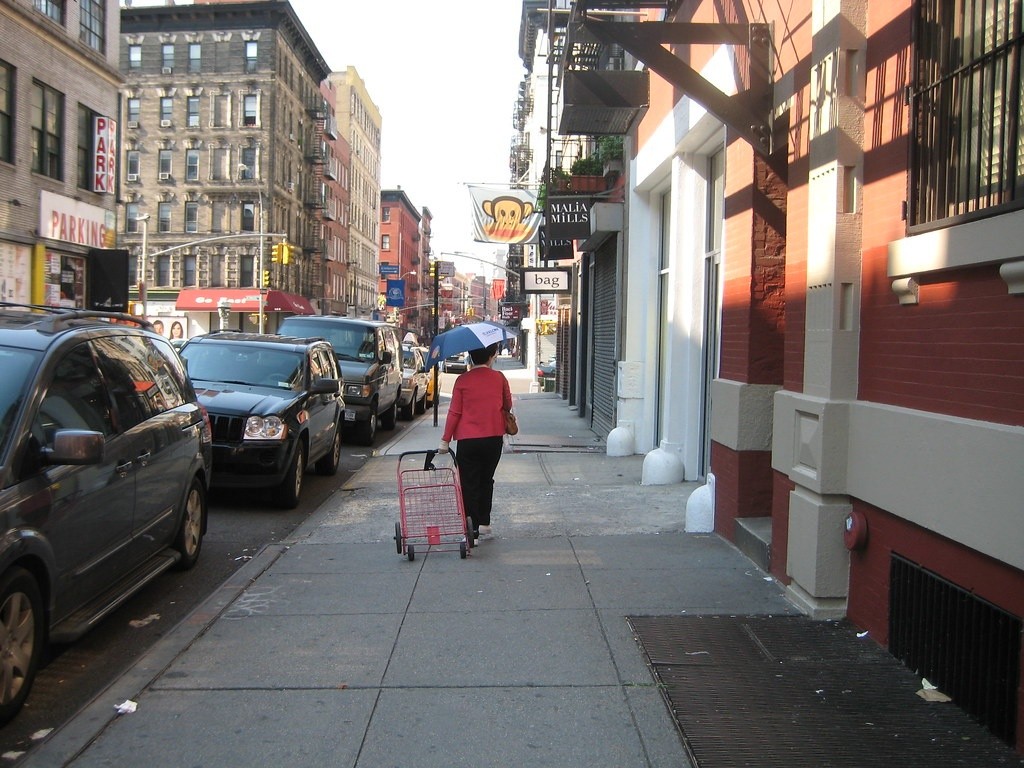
[438,343,513,546]
[153,320,183,339]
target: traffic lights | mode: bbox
[466,308,475,317]
[283,243,295,265]
[271,242,282,265]
[262,270,272,287]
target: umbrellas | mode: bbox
[425,322,519,372]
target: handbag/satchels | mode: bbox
[500,372,518,435]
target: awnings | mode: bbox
[175,288,315,314]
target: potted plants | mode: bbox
[538,135,623,209]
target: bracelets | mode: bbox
[440,441,449,447]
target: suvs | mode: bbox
[176,328,346,511]
[0,299,213,735]
[277,315,403,445]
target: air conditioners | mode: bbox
[162,67,172,75]
[290,134,295,141]
[128,174,139,182]
[128,121,139,128]
[161,120,172,127]
[160,173,170,180]
[288,182,294,190]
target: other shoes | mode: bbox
[462,537,478,546]
[479,525,492,535]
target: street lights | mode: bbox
[238,163,265,334]
[429,261,437,278]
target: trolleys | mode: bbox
[394,446,474,562]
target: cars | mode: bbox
[444,354,466,374]
[396,340,431,420]
[403,332,441,409]
[538,360,556,386]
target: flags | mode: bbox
[465,185,544,245]
